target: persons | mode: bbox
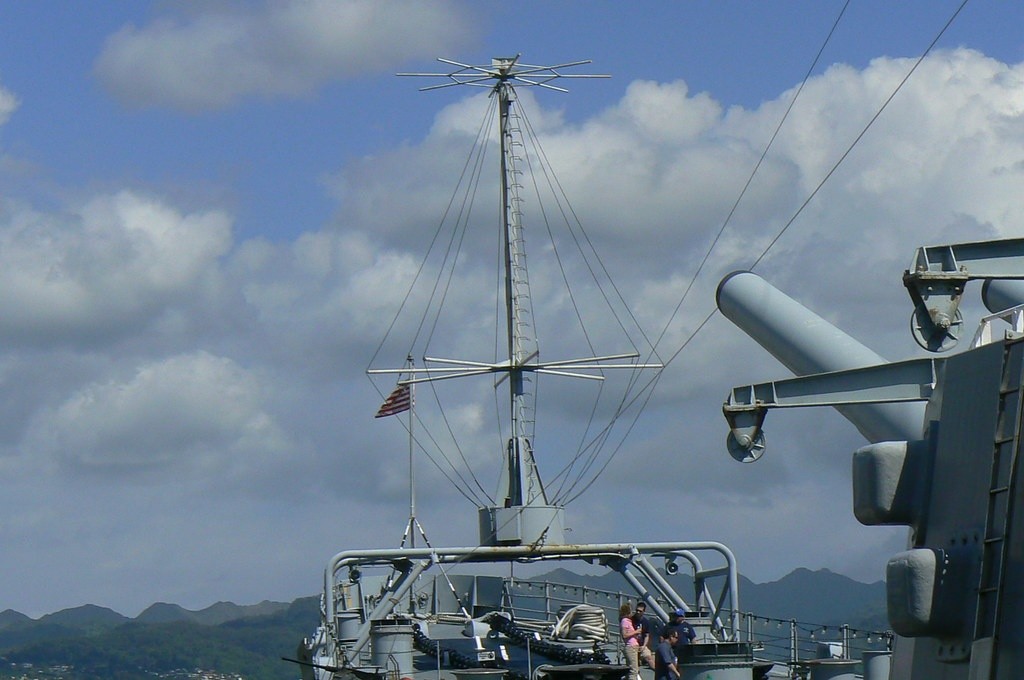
[655,608,696,680]
[618,603,642,680]
[632,602,655,680]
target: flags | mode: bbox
[375,378,415,418]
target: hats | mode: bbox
[674,609,685,617]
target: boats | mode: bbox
[295,52,1024,679]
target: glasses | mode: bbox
[636,608,644,612]
